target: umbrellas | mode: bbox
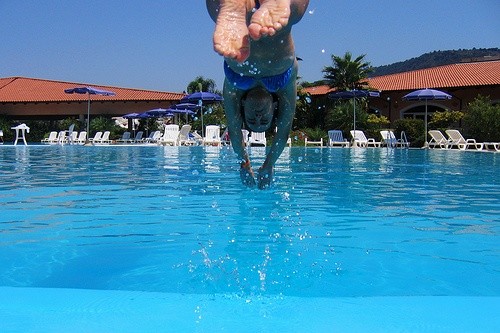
[401,87,452,146]
[180,91,224,137]
[64,87,115,139]
[328,90,380,140]
[123,103,205,138]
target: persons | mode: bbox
[206,0,309,191]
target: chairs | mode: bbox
[305,130,410,148]
[428,130,500,153]
[40,124,291,148]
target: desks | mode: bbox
[11,127,30,145]
